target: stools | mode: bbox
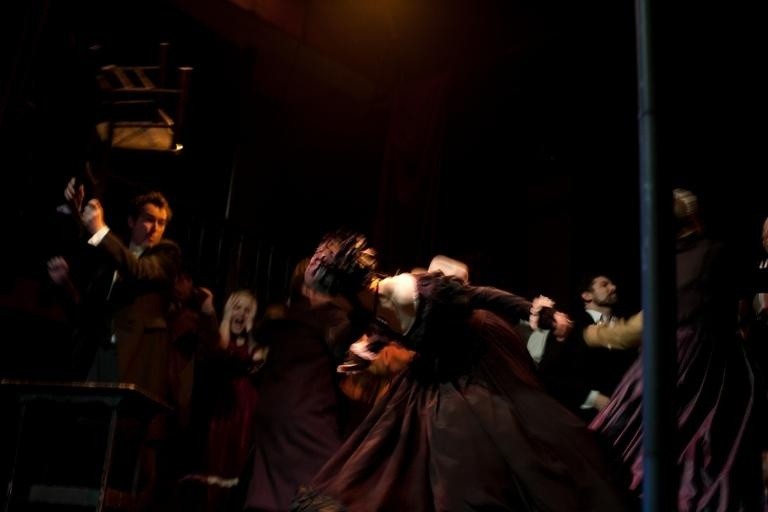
[1,378,174,511]
